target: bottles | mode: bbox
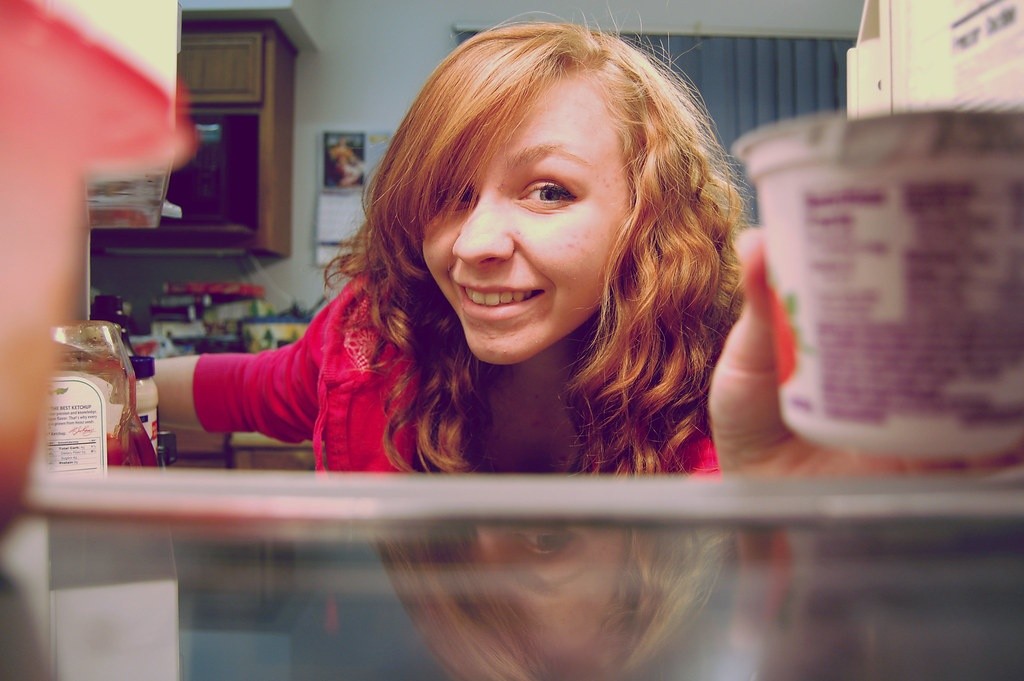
[90,293,136,359]
[127,357,159,459]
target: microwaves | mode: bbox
[97,114,257,245]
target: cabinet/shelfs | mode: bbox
[91,18,299,259]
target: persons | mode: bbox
[329,137,362,184]
[152,21,1024,472]
[371,520,735,681]
